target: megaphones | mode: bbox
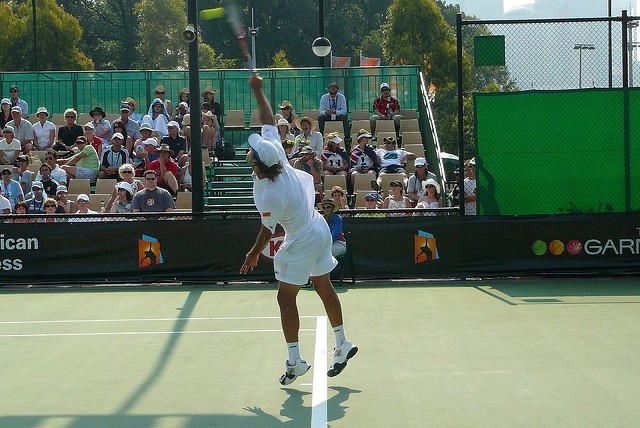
[181,24,197,43]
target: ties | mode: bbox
[332,99,337,108]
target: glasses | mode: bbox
[45,157,52,160]
[122,111,128,113]
[156,92,164,95]
[46,205,56,208]
[391,185,400,187]
[85,128,92,131]
[146,178,155,180]
[42,169,48,171]
[78,201,87,204]
[115,126,122,128]
[425,185,435,188]
[384,142,393,144]
[3,131,12,133]
[93,113,101,116]
[322,206,333,209]
[329,91,338,94]
[10,90,18,93]
[32,188,42,191]
[65,116,75,118]
[2,172,12,175]
[76,142,84,144]
[118,188,125,191]
[366,199,376,201]
[122,171,132,174]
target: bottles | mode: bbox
[100,198,105,213]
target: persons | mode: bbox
[239,72,359,386]
[0,186,12,214]
[470,157,476,194]
[7,201,37,223]
[330,185,350,217]
[176,88,189,108]
[369,82,402,138]
[413,178,443,216]
[160,120,186,159]
[320,131,349,176]
[84,122,102,159]
[5,106,34,145]
[8,85,28,118]
[142,138,159,162]
[169,102,190,130]
[153,85,172,115]
[134,123,160,148]
[37,197,66,222]
[356,193,386,217]
[276,118,295,141]
[281,138,295,159]
[36,150,67,185]
[24,182,46,213]
[201,111,217,149]
[32,107,56,150]
[131,169,176,221]
[61,136,100,184]
[370,135,417,194]
[278,100,298,129]
[182,114,191,138]
[201,102,220,147]
[84,107,112,139]
[406,157,438,201]
[118,96,143,124]
[349,129,378,207]
[382,180,412,217]
[0,98,13,123]
[293,145,323,202]
[0,125,22,165]
[0,168,25,213]
[317,82,351,138]
[39,162,60,194]
[179,151,206,190]
[464,159,476,216]
[295,116,323,159]
[55,185,77,213]
[301,196,347,287]
[103,162,146,213]
[12,155,32,195]
[136,145,150,162]
[68,193,102,222]
[202,86,220,124]
[109,104,141,140]
[142,98,169,136]
[57,108,84,148]
[108,119,132,152]
[110,181,134,212]
[98,132,130,179]
[292,138,311,158]
[147,143,178,196]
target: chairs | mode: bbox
[377,131,398,145]
[68,179,92,196]
[325,190,348,205]
[355,189,378,209]
[354,174,377,192]
[324,174,347,189]
[324,121,344,135]
[28,114,39,124]
[399,120,421,136]
[375,120,395,137]
[176,191,193,213]
[78,112,93,126]
[250,109,265,129]
[381,173,403,191]
[222,108,245,131]
[351,110,370,120]
[350,120,370,137]
[302,110,318,120]
[310,228,358,286]
[133,177,147,189]
[401,132,423,149]
[64,193,82,214]
[89,193,111,212]
[107,114,118,123]
[53,113,67,129]
[95,180,117,193]
[28,164,46,180]
[202,147,211,168]
[405,144,425,162]
[31,150,47,162]
[0,165,15,173]
[400,109,418,119]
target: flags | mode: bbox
[360,50,381,66]
[330,51,352,67]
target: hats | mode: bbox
[414,156,428,167]
[278,101,293,112]
[89,107,106,118]
[357,131,373,142]
[140,124,155,131]
[201,102,211,110]
[120,104,130,112]
[155,144,174,156]
[181,114,191,125]
[12,106,21,113]
[276,119,291,130]
[4,126,14,135]
[22,172,32,183]
[201,86,216,97]
[300,146,312,154]
[179,102,188,110]
[422,179,440,194]
[85,123,94,129]
[155,87,165,93]
[317,196,339,212]
[111,132,124,140]
[76,136,86,143]
[383,137,396,144]
[122,97,137,109]
[179,89,189,96]
[35,107,49,120]
[328,134,343,144]
[247,133,280,170]
[10,86,19,92]
[202,111,216,126]
[142,138,157,147]
[1,98,11,105]
[56,186,68,194]
[390,180,403,190]
[380,83,389,91]
[77,194,89,202]
[15,155,29,162]
[115,182,133,195]
[165,121,180,132]
[32,182,42,189]
[364,193,378,199]
[295,116,317,131]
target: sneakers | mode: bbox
[327,339,358,377]
[371,179,380,191]
[279,357,311,385]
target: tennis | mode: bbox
[201,8,223,21]
